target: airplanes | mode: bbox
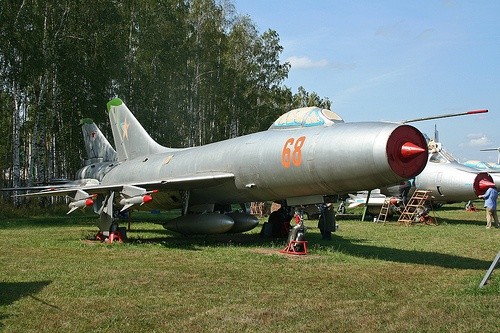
[1,97,489,244]
[333,123,496,221]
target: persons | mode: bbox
[399,181,411,205]
[478,187,500,228]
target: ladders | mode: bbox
[376,197,391,225]
[397,188,438,226]
